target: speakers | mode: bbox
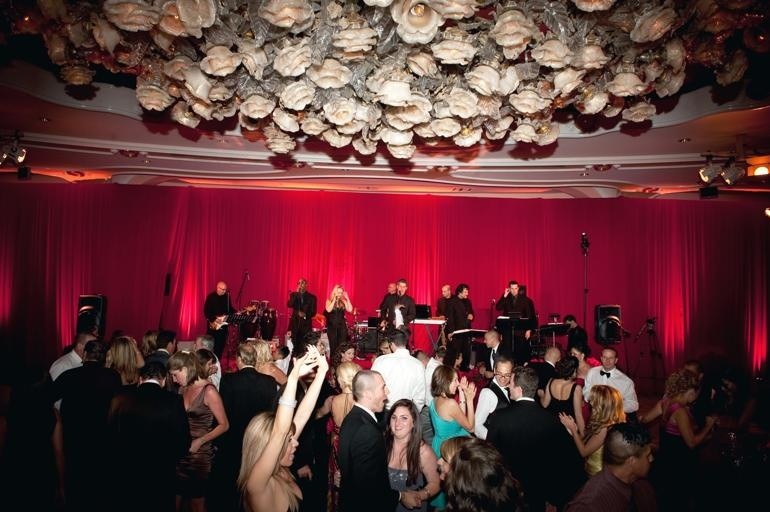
[77,295,107,339]
[595,305,621,344]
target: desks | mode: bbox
[405,318,447,356]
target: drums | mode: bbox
[354,324,368,333]
[240,310,258,339]
[251,301,259,309]
[261,301,269,309]
[261,308,276,340]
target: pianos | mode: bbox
[409,319,447,324]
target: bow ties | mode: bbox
[599,370,610,378]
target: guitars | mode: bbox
[207,310,246,331]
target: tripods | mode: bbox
[632,319,666,397]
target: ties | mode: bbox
[505,388,513,403]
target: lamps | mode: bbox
[0,127,27,165]
[693,151,745,185]
[12,0,769,161]
